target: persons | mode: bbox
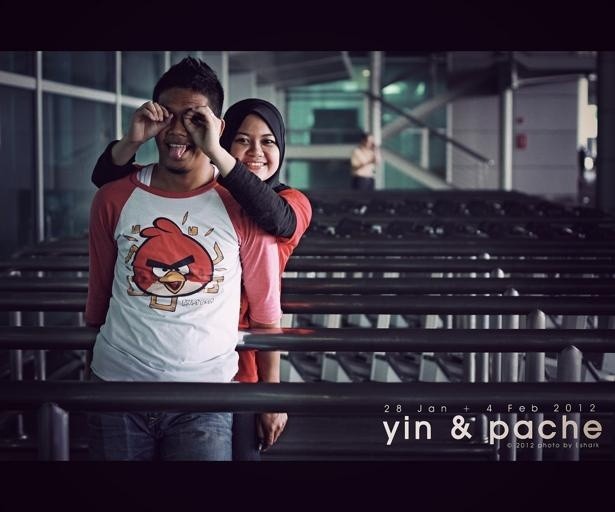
[90,99,313,462]
[83,57,289,462]
[350,132,379,193]
